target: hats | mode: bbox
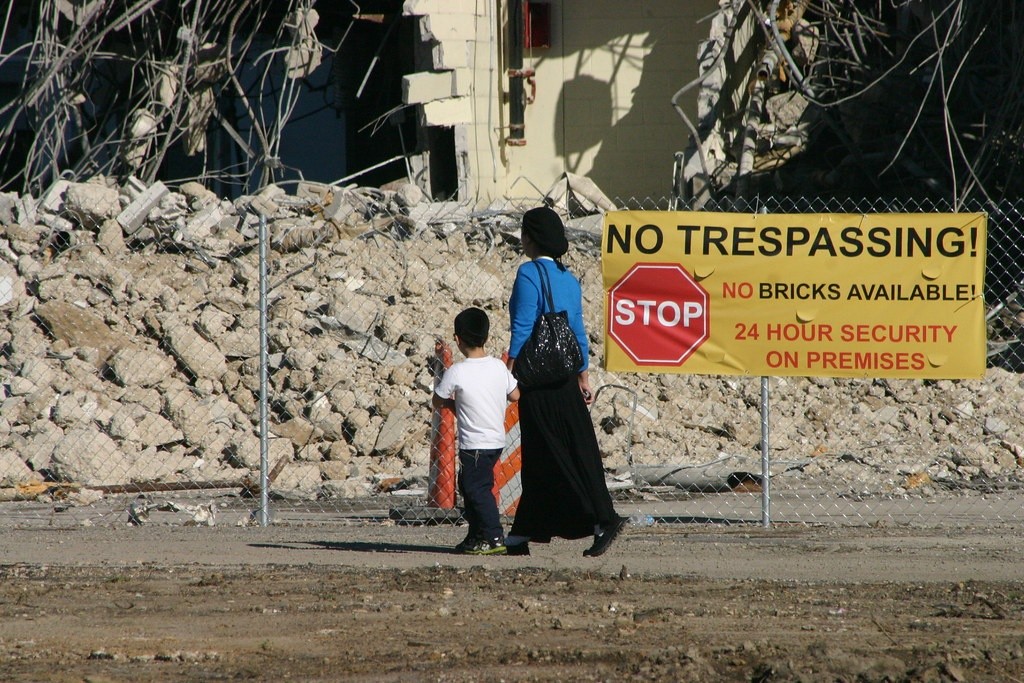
[521,207,569,257]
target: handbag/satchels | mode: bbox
[512,261,585,387]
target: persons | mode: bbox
[502,207,631,558]
[432,307,520,556]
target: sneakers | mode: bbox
[455,532,483,553]
[464,536,507,555]
[583,513,630,556]
[503,541,530,555]
